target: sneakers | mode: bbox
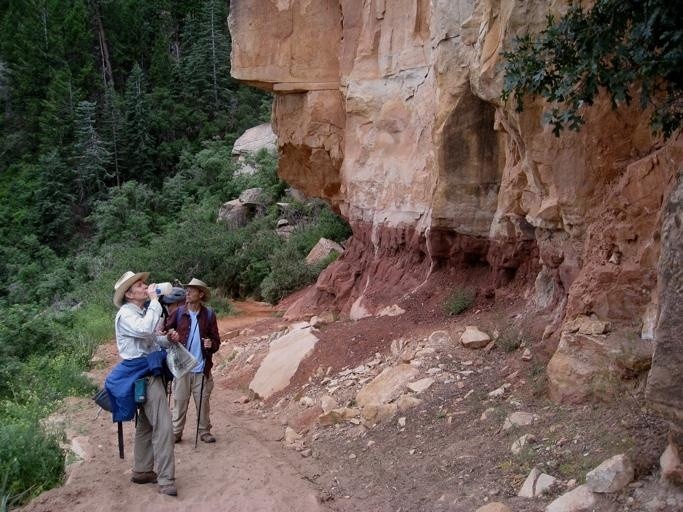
[131,470,158,484]
[173,433,181,444]
[199,432,216,443]
[158,479,177,496]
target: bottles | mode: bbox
[146,281,173,297]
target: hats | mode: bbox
[179,277,212,302]
[162,286,186,304]
[111,269,150,309]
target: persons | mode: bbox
[110,270,181,496]
[161,277,220,442]
[158,285,185,323]
